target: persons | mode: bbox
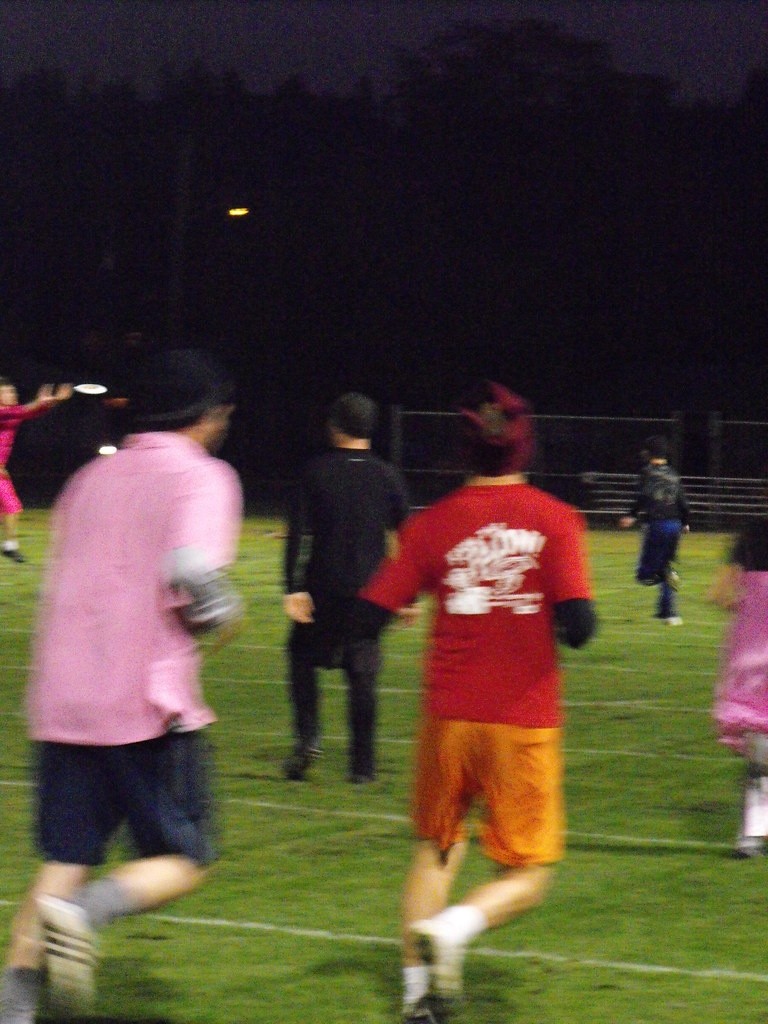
[710,519,768,857]
[71,379,130,463]
[0,377,76,563]
[352,377,597,1024]
[617,432,691,625]
[278,390,419,785]
[0,349,247,1024]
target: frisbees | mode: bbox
[99,446,117,454]
[74,383,108,395]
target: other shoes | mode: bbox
[285,735,320,775]
[410,919,464,1017]
[350,770,376,786]
[36,889,97,1013]
[2,548,26,563]
[402,998,437,1024]
